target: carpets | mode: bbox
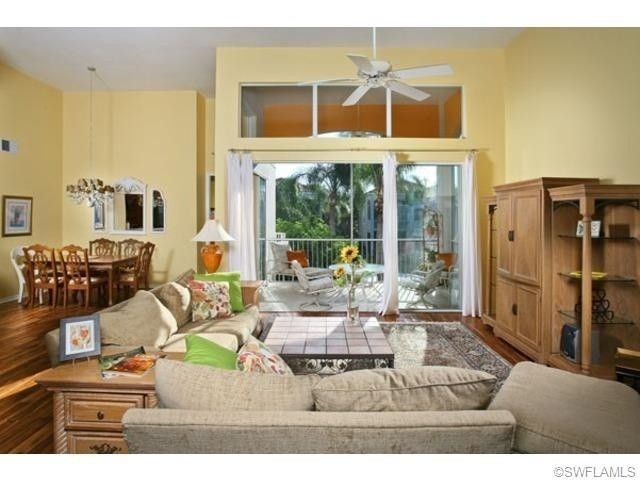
[256,321,514,411]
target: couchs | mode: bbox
[44,276,265,366]
[121,404,516,454]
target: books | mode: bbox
[98,355,163,380]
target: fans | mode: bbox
[295,27,453,107]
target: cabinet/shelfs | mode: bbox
[35,347,185,454]
[480,176,640,381]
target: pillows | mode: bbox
[98,267,246,350]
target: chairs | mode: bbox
[398,260,447,309]
[265,241,334,312]
[10,237,155,312]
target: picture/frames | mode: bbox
[2,194,34,237]
[59,314,101,362]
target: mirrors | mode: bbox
[92,176,165,235]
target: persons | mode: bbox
[10,213,24,226]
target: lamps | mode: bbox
[189,219,236,274]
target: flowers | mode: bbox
[329,237,368,321]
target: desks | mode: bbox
[328,262,384,303]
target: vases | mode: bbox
[346,288,360,326]
